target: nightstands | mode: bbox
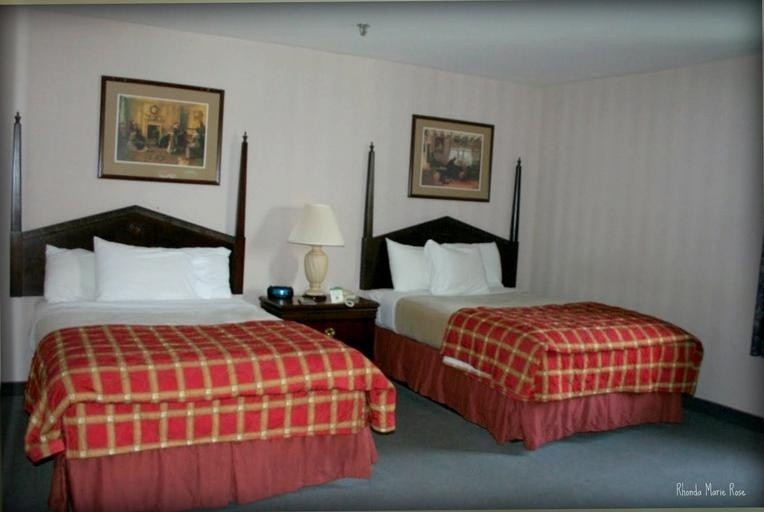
[259,294,380,363]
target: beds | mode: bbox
[357,141,704,453]
[8,111,396,512]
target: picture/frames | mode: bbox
[408,114,494,202]
[97,75,225,185]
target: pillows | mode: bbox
[41,235,233,303]
[384,237,504,298]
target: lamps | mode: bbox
[286,202,344,298]
